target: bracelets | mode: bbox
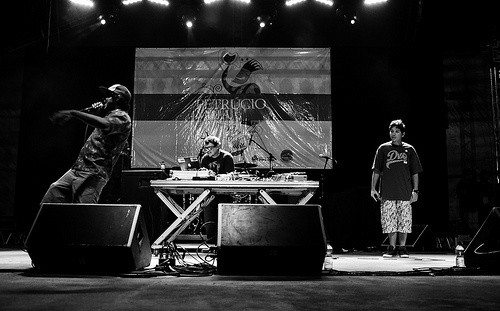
[412,189,420,193]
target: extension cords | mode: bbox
[159,251,175,266]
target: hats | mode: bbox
[99,83,131,105]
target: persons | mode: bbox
[201,135,234,244]
[371,120,423,258]
[40,84,131,209]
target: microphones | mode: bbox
[249,135,253,145]
[81,104,103,112]
[319,154,337,162]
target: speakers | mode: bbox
[463,208,500,268]
[120,169,172,242]
[24,203,151,274]
[216,203,327,276]
[381,223,437,253]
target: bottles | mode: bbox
[161,161,165,170]
[455,241,465,267]
[323,241,333,270]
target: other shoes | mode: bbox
[383,245,410,258]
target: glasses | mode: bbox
[205,147,214,153]
